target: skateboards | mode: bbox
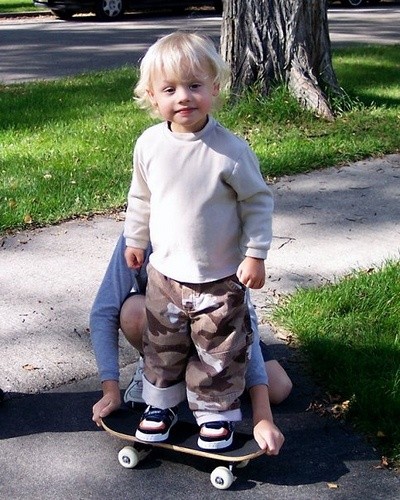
[100,403,266,490]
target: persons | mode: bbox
[90,233,294,457]
[122,30,275,450]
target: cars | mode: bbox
[330,0,394,8]
[45,0,188,18]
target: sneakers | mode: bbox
[124,366,144,402]
[197,422,236,449]
[135,405,178,442]
